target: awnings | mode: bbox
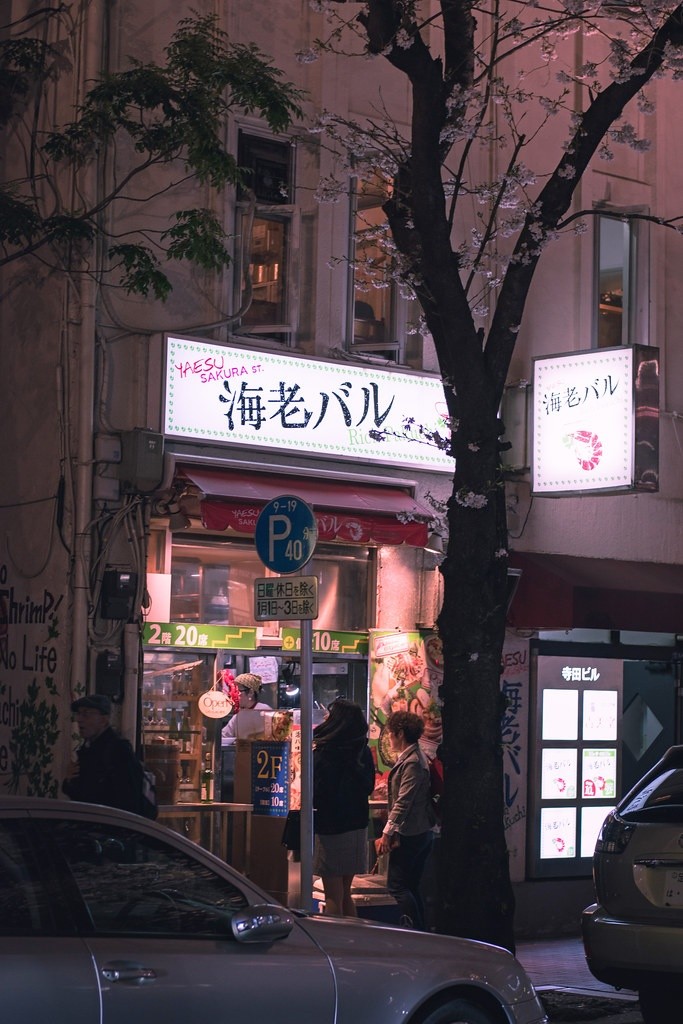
[507,552,683,637]
[176,464,433,546]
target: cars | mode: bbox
[0,794,548,1024]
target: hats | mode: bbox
[70,694,111,715]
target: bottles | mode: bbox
[141,706,191,754]
[199,759,215,804]
[147,759,192,802]
[171,670,185,696]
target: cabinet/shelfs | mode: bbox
[144,662,204,801]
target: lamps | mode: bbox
[425,525,445,555]
[157,500,192,533]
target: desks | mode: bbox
[151,802,254,883]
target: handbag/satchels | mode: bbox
[280,809,317,850]
[427,753,444,795]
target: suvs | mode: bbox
[582,744,683,1024]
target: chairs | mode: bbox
[54,867,99,937]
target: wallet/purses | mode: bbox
[371,833,400,874]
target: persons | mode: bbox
[312,699,375,917]
[222,674,274,746]
[61,693,142,864]
[375,710,435,933]
